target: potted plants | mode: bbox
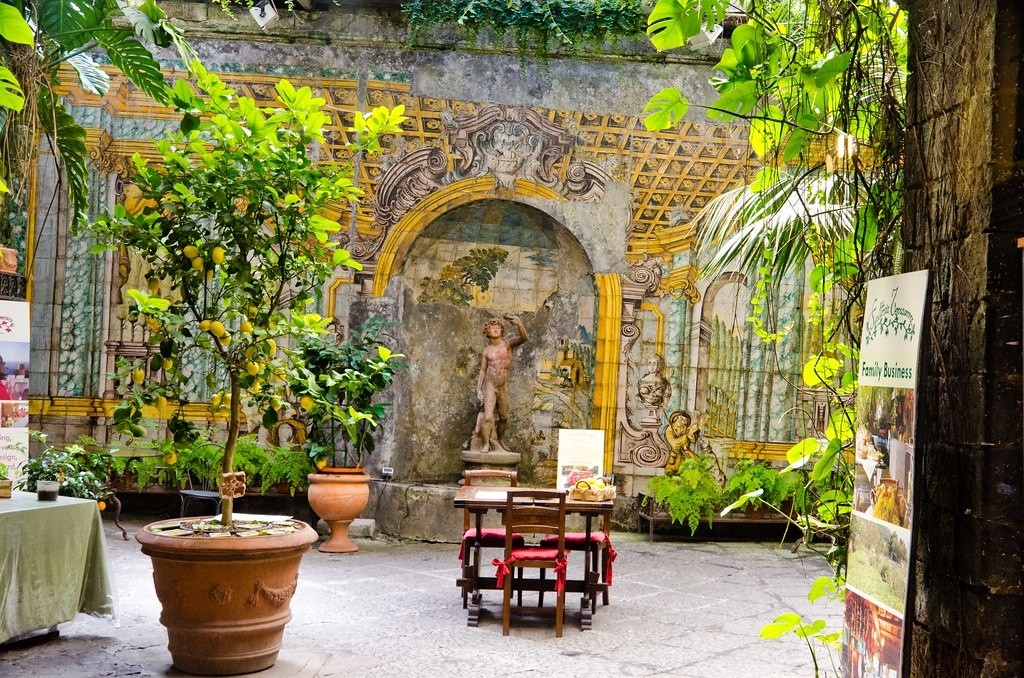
[639,456,845,544]
[259,442,316,498]
[65,64,406,676]
[0,462,14,498]
[291,314,408,552]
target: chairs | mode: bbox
[458,470,524,609]
[538,470,617,608]
[492,490,569,638]
[177,443,226,518]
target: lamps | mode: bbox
[686,21,724,51]
[247,0,279,34]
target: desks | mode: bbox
[54,445,163,541]
[453,485,614,632]
[0,491,121,654]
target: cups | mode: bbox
[37,480,60,501]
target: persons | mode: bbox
[0,355,29,400]
[477,315,529,453]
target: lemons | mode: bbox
[165,452,176,464]
[323,458,326,466]
[578,482,588,488]
[588,479,595,484]
[598,484,605,488]
[316,461,323,470]
[132,246,313,415]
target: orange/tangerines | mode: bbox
[98,501,106,510]
